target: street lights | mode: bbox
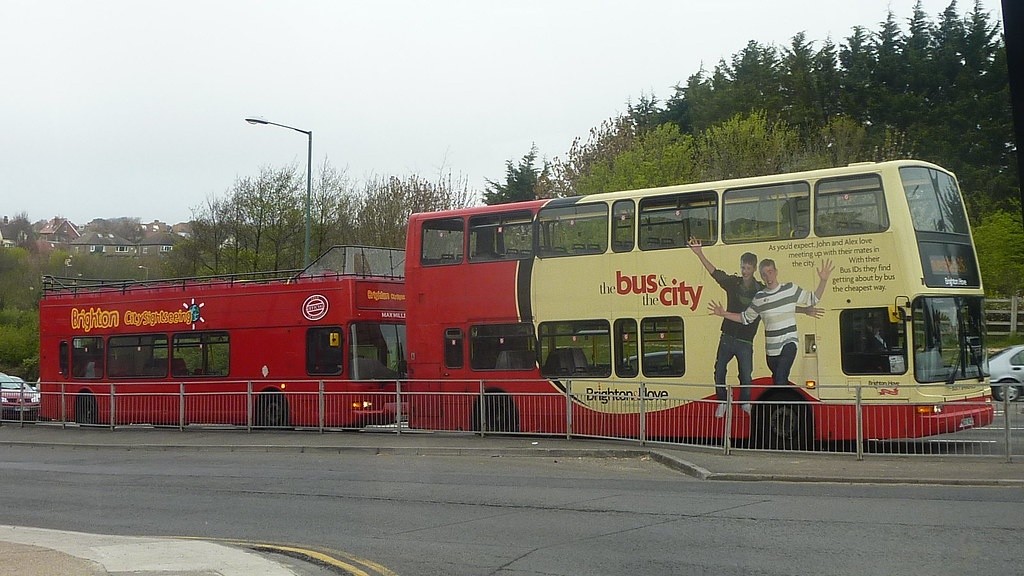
[245,117,312,274]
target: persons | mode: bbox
[706,258,837,395]
[686,235,825,427]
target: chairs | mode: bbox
[439,238,674,266]
[45,275,384,299]
[497,346,592,374]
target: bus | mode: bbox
[38,271,410,436]
[404,158,995,451]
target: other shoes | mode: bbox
[715,403,726,419]
[741,404,752,416]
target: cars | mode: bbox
[0,371,40,420]
[983,344,1024,401]
